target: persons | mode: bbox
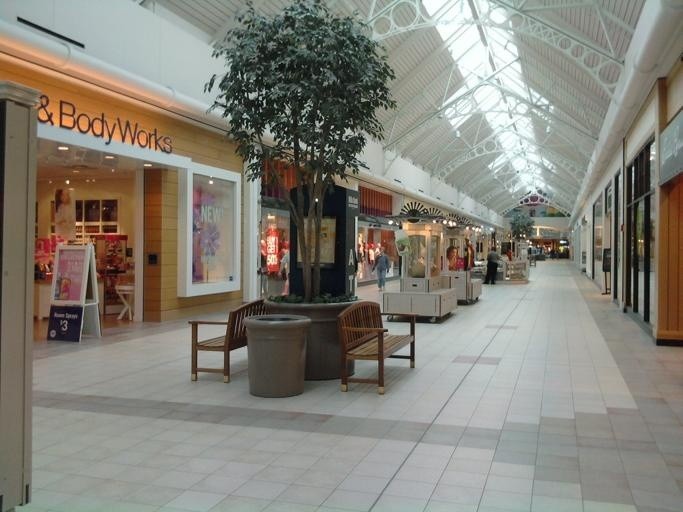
[549,248,560,260]
[482,247,500,284]
[277,251,289,295]
[371,249,389,291]
[528,244,536,267]
[55,188,75,241]
[260,240,270,298]
[358,242,381,280]
[446,247,459,271]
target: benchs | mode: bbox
[187,296,271,384]
[334,299,418,395]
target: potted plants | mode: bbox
[202,0,402,383]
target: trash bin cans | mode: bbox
[243,313,311,398]
